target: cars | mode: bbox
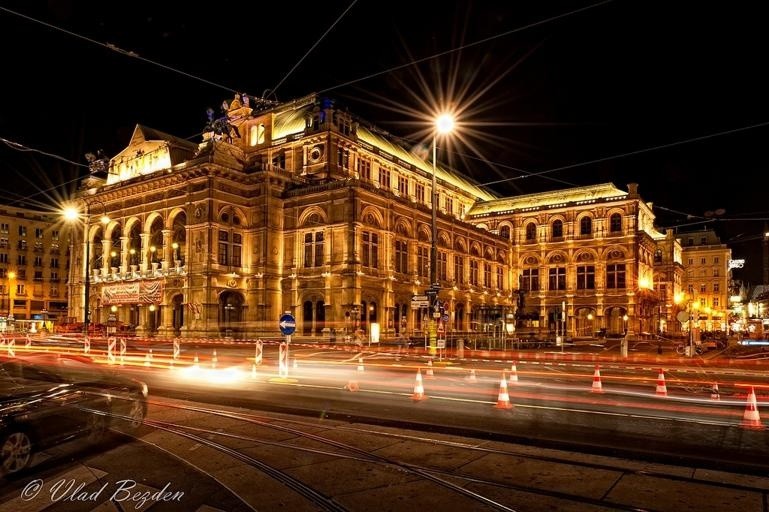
[0,349,153,494]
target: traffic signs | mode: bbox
[411,294,428,301]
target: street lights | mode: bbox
[427,112,456,316]
[675,293,695,361]
[61,196,111,333]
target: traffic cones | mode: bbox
[257,352,723,410]
[136,345,223,375]
[738,382,766,432]
